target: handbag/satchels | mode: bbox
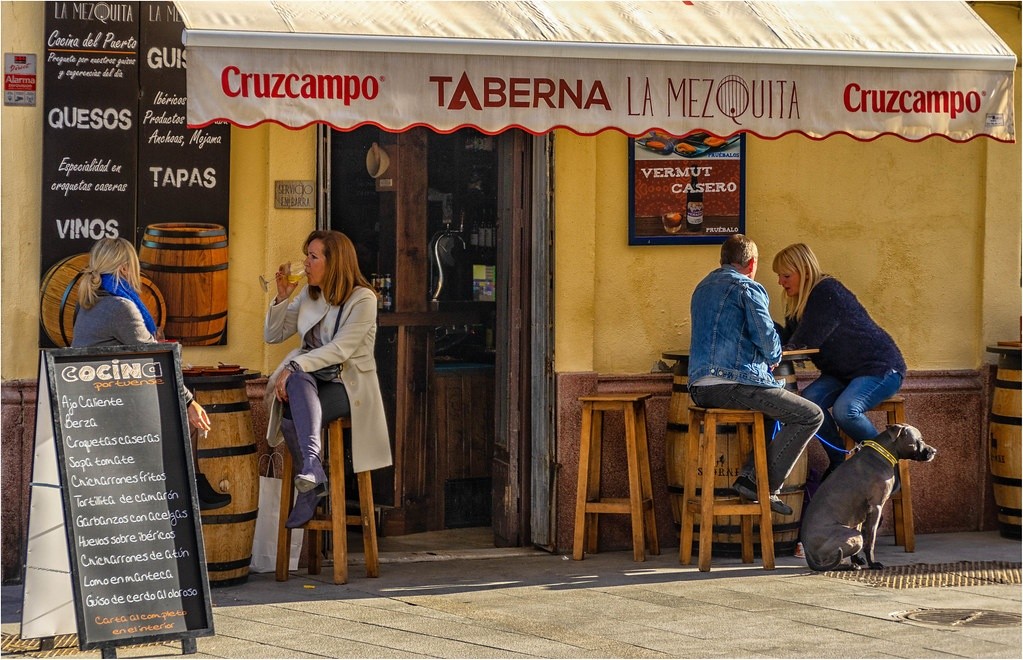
[313,364,340,381]
[250,453,307,574]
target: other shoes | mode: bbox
[733,476,793,515]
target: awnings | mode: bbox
[172,0,1017,144]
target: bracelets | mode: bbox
[285,365,294,372]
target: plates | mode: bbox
[636,136,673,156]
[673,140,711,157]
[180,369,201,375]
[685,132,740,150]
[203,367,249,375]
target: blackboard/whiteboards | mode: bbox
[20,341,216,651]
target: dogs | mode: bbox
[800,423,937,571]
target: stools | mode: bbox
[275,417,380,585]
[572,393,660,562]
[839,396,915,553]
[678,406,776,572]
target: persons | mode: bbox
[687,234,824,515]
[772,241,905,495]
[263,229,392,529]
[72,238,231,511]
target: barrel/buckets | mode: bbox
[140,223,229,346]
[668,360,808,552]
[40,252,166,348]
[988,353,1022,540]
[182,382,259,587]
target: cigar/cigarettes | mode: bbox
[205,430,207,438]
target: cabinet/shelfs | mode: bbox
[377,130,495,313]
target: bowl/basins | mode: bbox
[218,365,240,370]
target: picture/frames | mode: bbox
[628,129,745,246]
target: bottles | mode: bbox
[686,165,704,232]
[466,204,497,249]
[370,272,393,310]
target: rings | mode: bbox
[280,389,282,391]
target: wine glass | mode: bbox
[260,266,308,291]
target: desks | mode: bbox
[986,345,1022,541]
[660,348,819,560]
[185,369,269,589]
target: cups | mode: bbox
[661,204,683,234]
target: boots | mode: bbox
[892,466,900,495]
[286,371,329,498]
[195,473,232,508]
[802,441,846,490]
[281,417,330,529]
